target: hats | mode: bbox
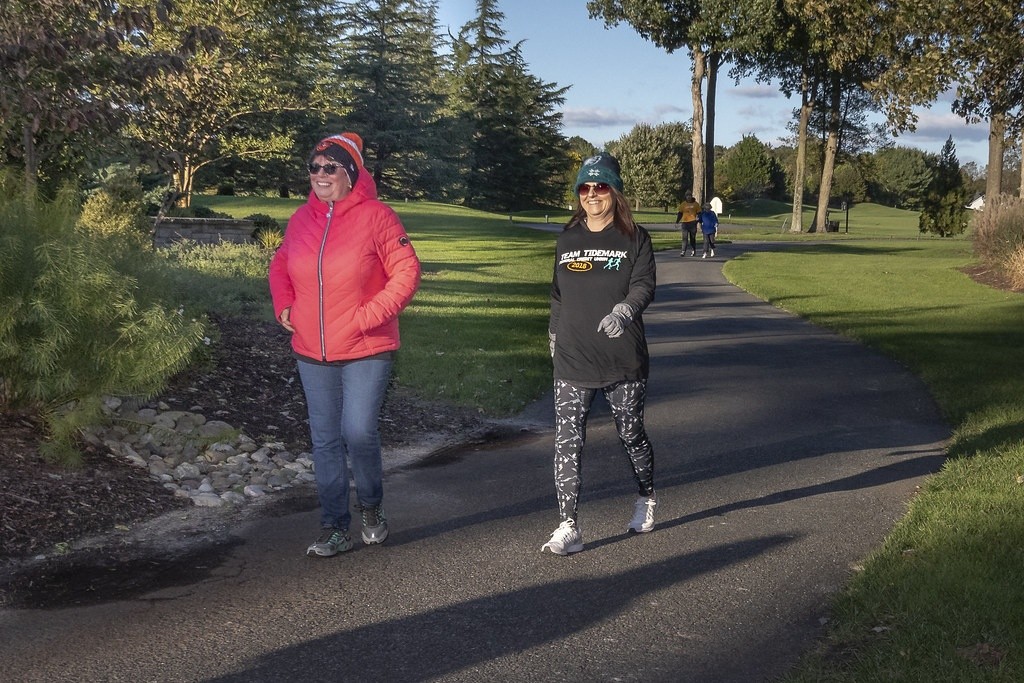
[575,152,623,198]
[309,132,363,191]
[684,189,692,196]
[704,202,712,208]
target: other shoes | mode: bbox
[691,249,696,256]
[680,251,685,256]
[701,253,707,258]
[710,248,714,257]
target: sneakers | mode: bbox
[541,519,584,556]
[358,500,389,545]
[626,488,659,533]
[306,527,354,558]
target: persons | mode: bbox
[699,203,719,258]
[269,132,420,555]
[540,153,659,554]
[675,189,702,256]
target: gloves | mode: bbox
[597,303,634,339]
[548,328,556,358]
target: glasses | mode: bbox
[579,182,611,195]
[308,162,344,175]
